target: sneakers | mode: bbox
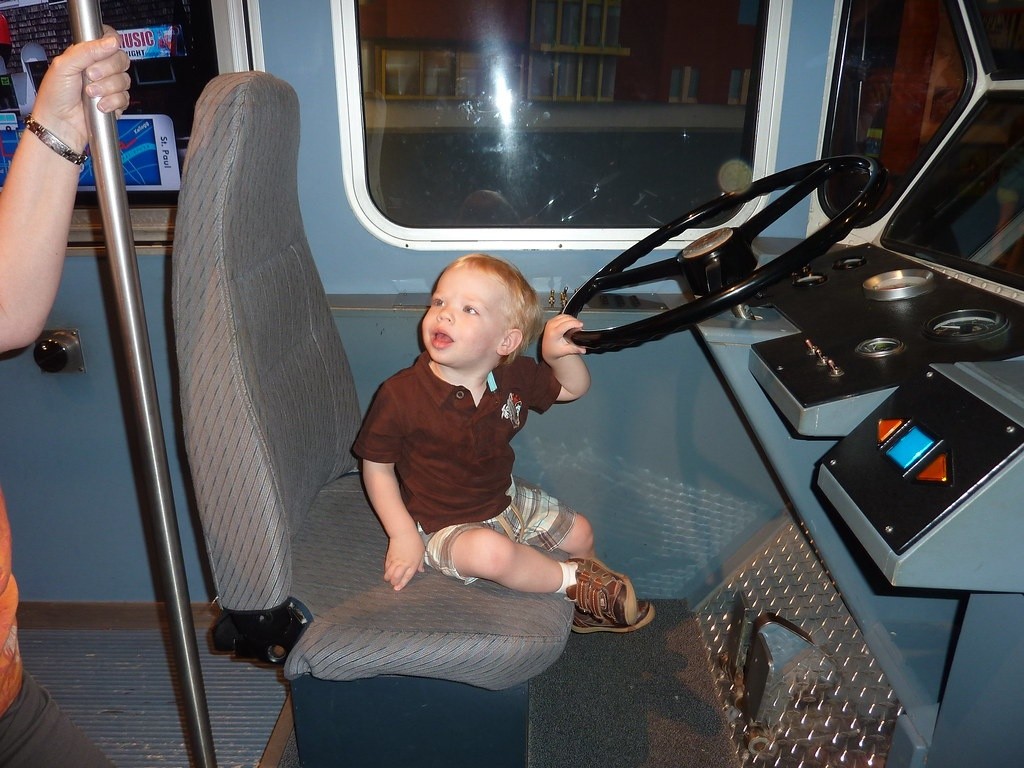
[564,557,655,634]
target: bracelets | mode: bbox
[23,113,89,166]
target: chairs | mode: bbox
[170,68,578,691]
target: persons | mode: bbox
[353,253,656,633]
[0,23,131,768]
